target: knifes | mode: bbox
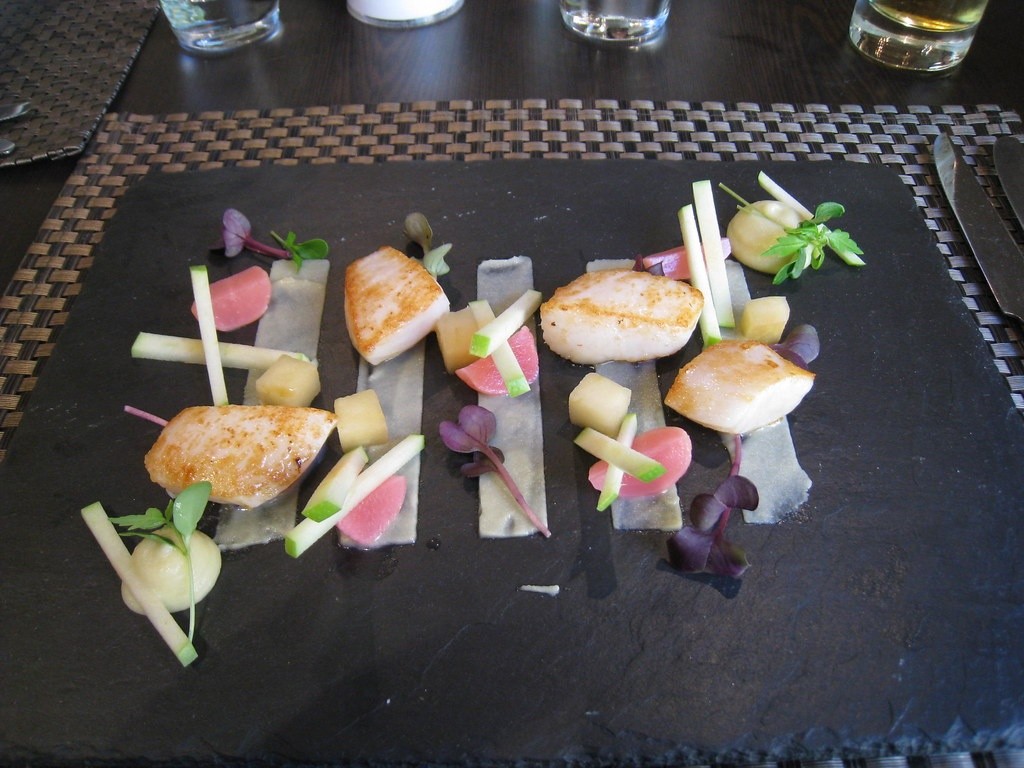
[993,136,1024,229]
[934,132,1024,321]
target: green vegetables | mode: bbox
[719,183,864,285]
[271,230,329,274]
[114,482,213,642]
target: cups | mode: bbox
[347,1,464,29]
[158,0,279,53]
[850,0,989,80]
[559,0,670,47]
